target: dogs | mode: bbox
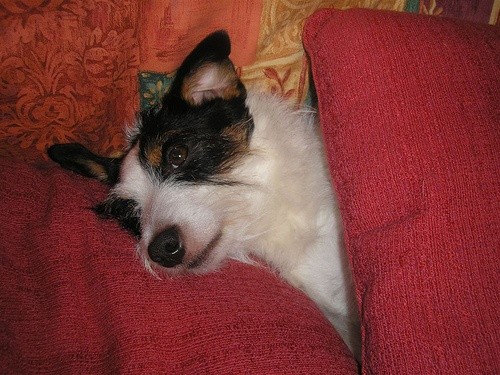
[45,29,364,365]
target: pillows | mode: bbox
[301,8,500,375]
[1,157,360,374]
